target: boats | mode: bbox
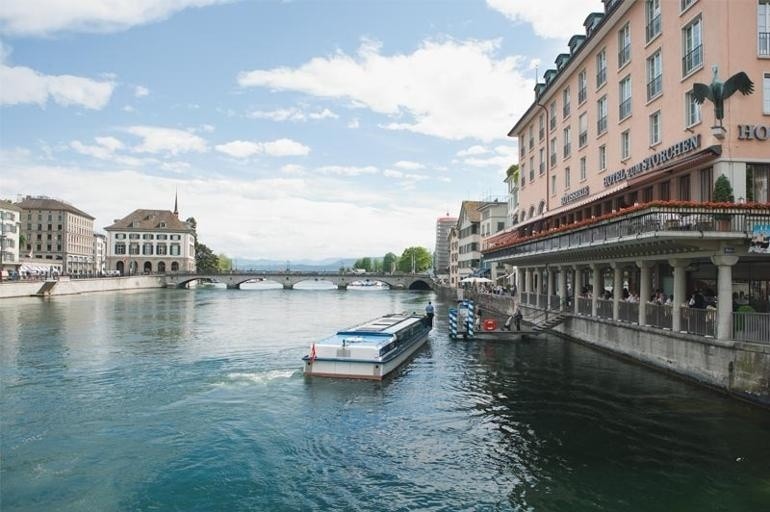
[301,310,438,382]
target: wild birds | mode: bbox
[692,64,755,127]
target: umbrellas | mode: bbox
[459,277,471,283]
[476,276,494,283]
[467,276,480,282]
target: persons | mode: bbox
[425,301,435,328]
[581,283,707,331]
[514,309,524,330]
[500,314,514,330]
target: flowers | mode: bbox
[652,199,770,210]
[480,201,652,256]
